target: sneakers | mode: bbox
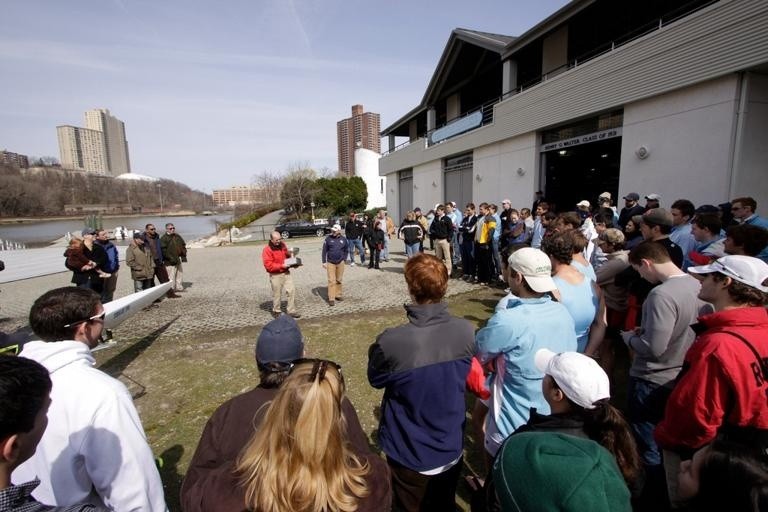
[99,272,112,278]
[327,297,343,306]
[458,273,512,294]
[275,311,301,319]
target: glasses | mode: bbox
[167,227,175,230]
[149,228,156,231]
[66,311,105,327]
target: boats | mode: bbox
[115,226,141,240]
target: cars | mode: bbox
[277,220,332,239]
[355,213,365,223]
[314,219,330,224]
[331,217,346,229]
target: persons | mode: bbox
[322,223,350,306]
[0,287,168,512]
[262,231,302,320]
[176,316,393,512]
[345,209,394,268]
[126,223,187,311]
[364,248,637,512]
[397,199,560,294]
[62,226,119,340]
[542,193,768,510]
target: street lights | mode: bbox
[71,187,75,200]
[126,190,132,204]
[157,183,163,212]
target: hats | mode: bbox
[134,232,145,238]
[330,224,342,231]
[502,199,512,204]
[591,229,624,246]
[623,192,639,201]
[508,248,557,294]
[82,228,96,235]
[688,255,768,292]
[576,200,591,207]
[493,432,632,511]
[633,208,673,226]
[535,348,611,409]
[599,192,611,200]
[255,317,304,370]
